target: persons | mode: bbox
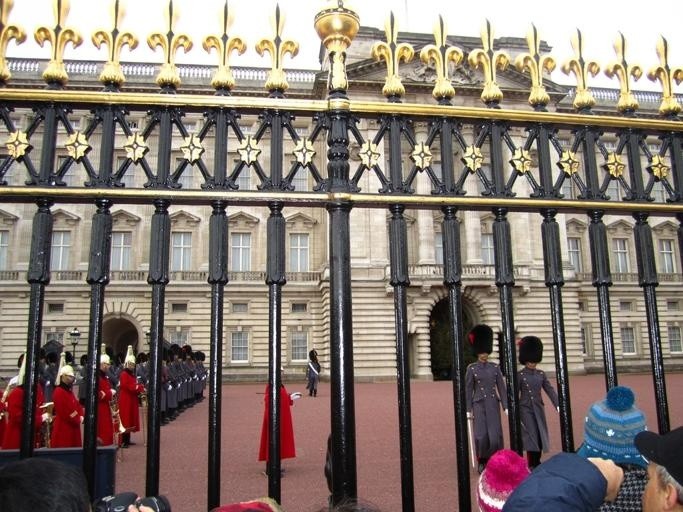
[575,386,651,512]
[500,425,683,512]
[0,457,156,512]
[0,344,208,449]
[258,383,295,478]
[464,353,508,474]
[517,361,559,470]
[306,359,321,397]
[309,348,317,362]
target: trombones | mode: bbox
[39,402,54,448]
[138,388,147,446]
[109,396,127,463]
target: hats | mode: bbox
[468,322,494,357]
[309,350,318,357]
[515,333,545,364]
[633,423,683,487]
[79,344,207,364]
[59,364,74,378]
[574,385,650,467]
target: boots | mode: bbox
[157,391,205,425]
